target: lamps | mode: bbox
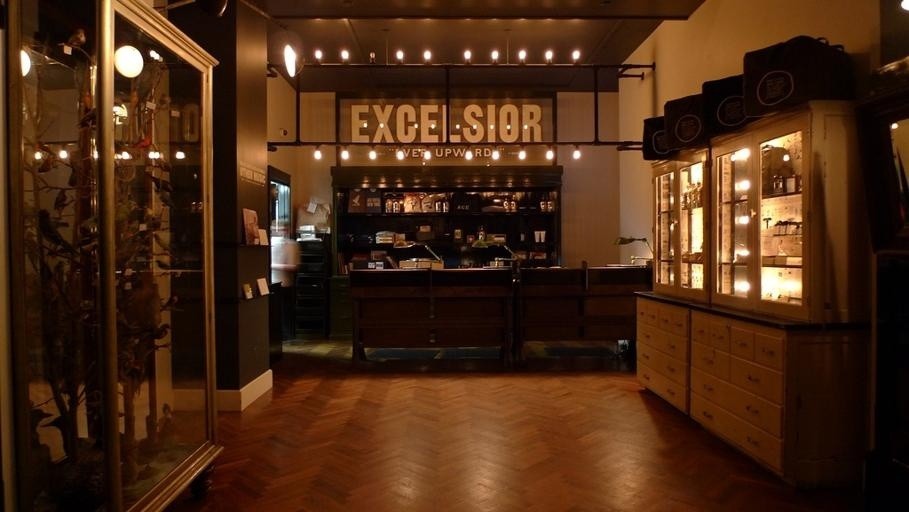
[114,45,144,78]
[616,224,651,265]
[27,142,189,164]
[306,46,585,69]
[307,143,584,161]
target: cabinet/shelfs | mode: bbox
[332,169,561,337]
[632,100,865,495]
[1,0,225,510]
[288,225,332,338]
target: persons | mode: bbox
[272,219,300,329]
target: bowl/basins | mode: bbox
[762,256,803,265]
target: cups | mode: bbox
[534,230,545,243]
[772,173,796,193]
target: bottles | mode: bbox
[478,225,486,241]
[682,182,703,207]
[504,194,517,212]
[385,199,400,213]
[435,199,450,212]
[540,195,555,211]
[775,221,803,235]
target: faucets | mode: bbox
[423,244,441,262]
[503,244,516,257]
